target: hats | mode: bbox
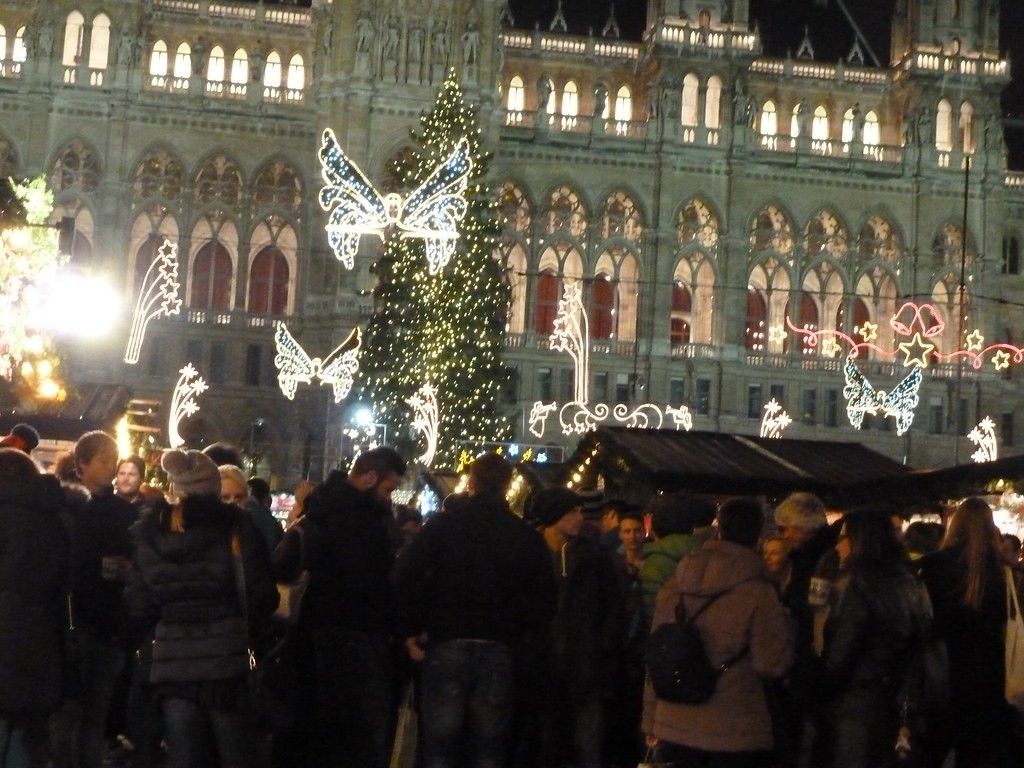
[530,486,583,526]
[0,424,40,456]
[774,492,826,532]
[576,485,606,519]
[161,449,221,499]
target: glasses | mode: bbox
[836,535,848,544]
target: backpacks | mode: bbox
[648,574,759,704]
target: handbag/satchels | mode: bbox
[247,635,295,705]
[892,572,956,761]
[1003,564,1024,715]
[637,743,674,768]
[271,526,308,625]
[388,678,418,768]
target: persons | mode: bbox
[0,422,1024,768]
